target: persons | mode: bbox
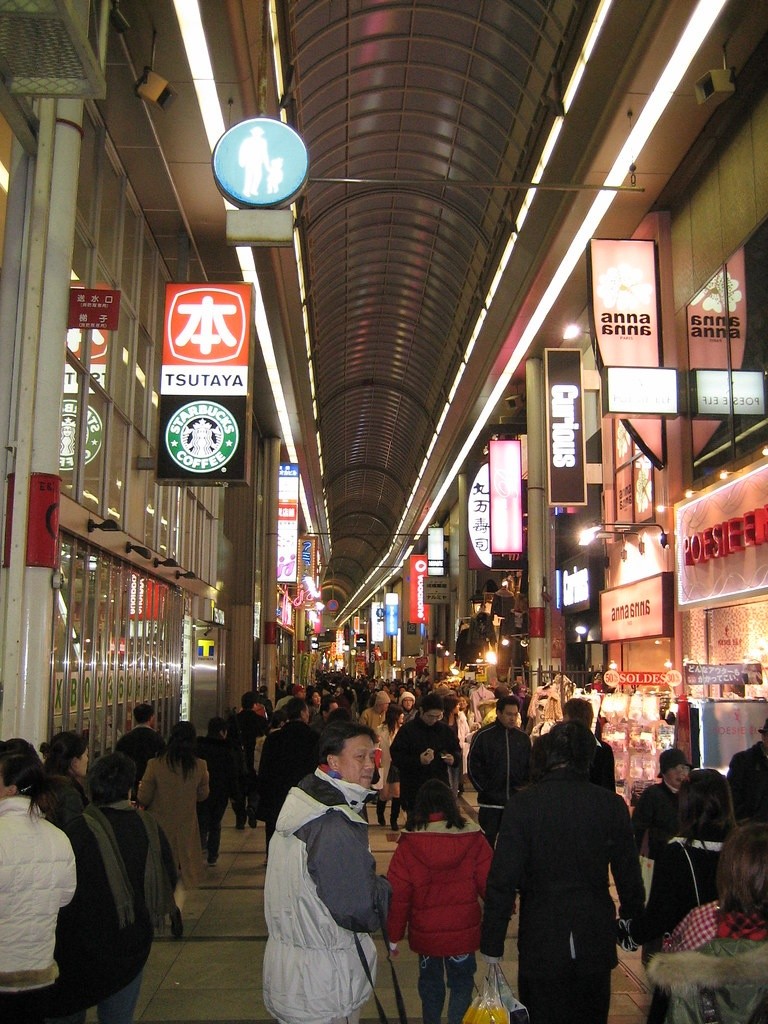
[138,722,210,936]
[480,721,649,1024]
[615,767,735,1023]
[198,716,238,866]
[262,720,391,1024]
[728,717,768,821]
[0,732,177,1024]
[651,821,767,1024]
[630,749,692,863]
[386,781,515,1024]
[226,670,693,940]
[116,704,167,801]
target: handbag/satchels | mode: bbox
[659,839,723,952]
[460,964,530,1024]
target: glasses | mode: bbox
[426,714,443,721]
[403,700,414,703]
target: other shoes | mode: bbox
[236,824,245,830]
[390,819,399,830]
[207,852,219,864]
[245,807,257,828]
[380,820,386,827]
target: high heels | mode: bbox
[170,907,184,936]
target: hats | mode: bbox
[657,749,693,778]
[375,691,391,704]
[757,718,768,733]
[400,691,415,705]
[292,684,306,696]
